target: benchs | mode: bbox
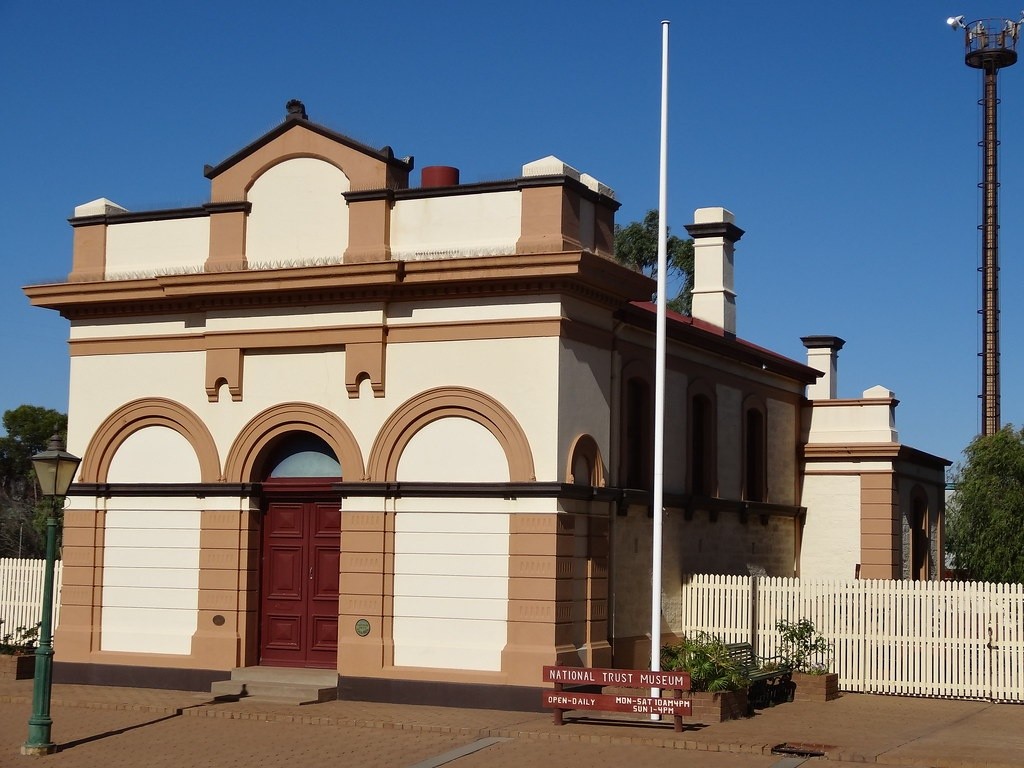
[721,642,794,716]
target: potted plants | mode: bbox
[646,630,753,722]
[766,617,839,701]
[0,617,42,680]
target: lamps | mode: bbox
[1003,19,1020,39]
[968,21,985,43]
[947,15,965,31]
